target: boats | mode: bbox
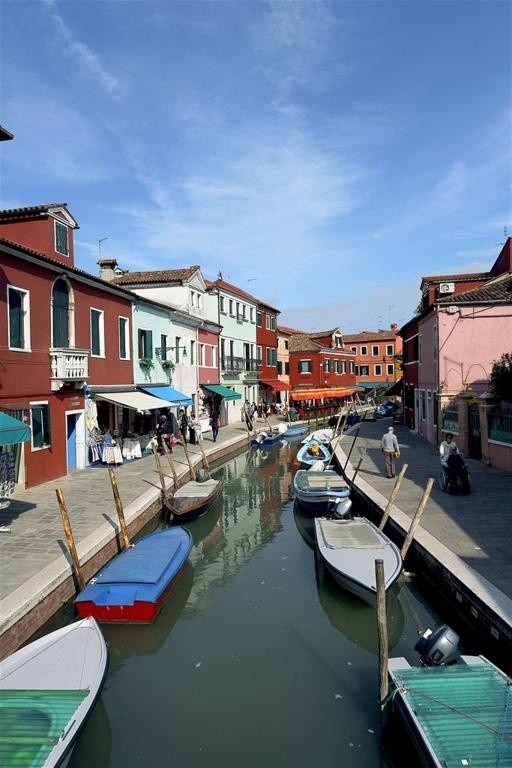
[385,623,511,768]
[167,493,224,551]
[250,400,401,470]
[164,469,225,520]
[312,498,404,609]
[314,550,406,656]
[99,558,194,656]
[292,496,354,551]
[0,616,109,768]
[293,468,352,508]
[74,523,196,628]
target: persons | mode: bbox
[209,411,221,444]
[156,405,200,455]
[380,424,401,479]
[438,432,460,460]
[441,444,469,494]
[240,397,300,432]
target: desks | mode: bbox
[102,437,158,467]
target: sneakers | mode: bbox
[387,474,395,479]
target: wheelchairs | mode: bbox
[437,458,469,491]
